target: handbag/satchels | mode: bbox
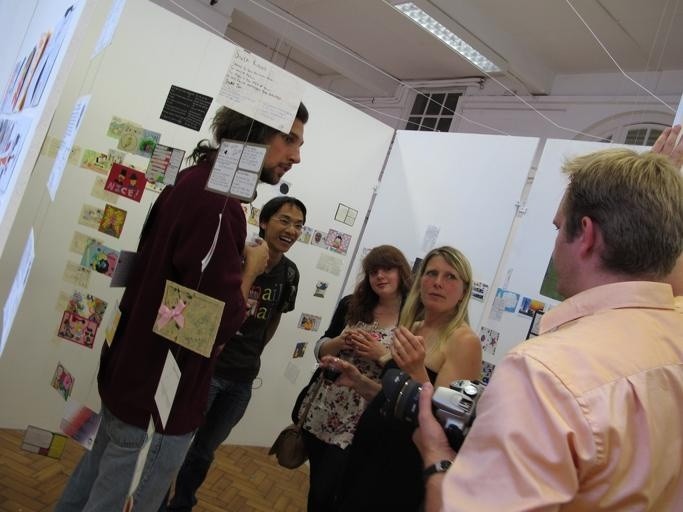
[268,422,309,470]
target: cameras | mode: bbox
[378,369,485,453]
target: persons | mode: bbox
[319,245,482,512]
[292,244,416,512]
[158,195,308,512]
[412,122,683,511]
[55,102,307,510]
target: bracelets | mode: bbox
[422,460,451,478]
[377,352,393,367]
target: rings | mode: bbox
[334,358,339,363]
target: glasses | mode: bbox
[271,217,303,233]
